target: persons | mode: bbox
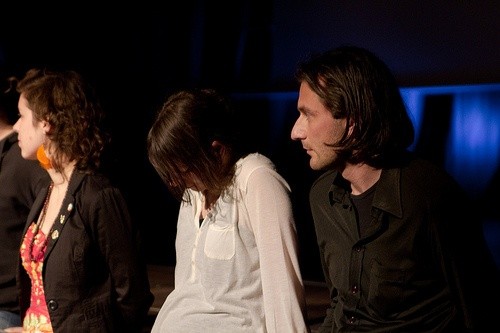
[289,42,500,333]
[146,87,308,333]
[0,66,49,329]
[11,62,155,333]
